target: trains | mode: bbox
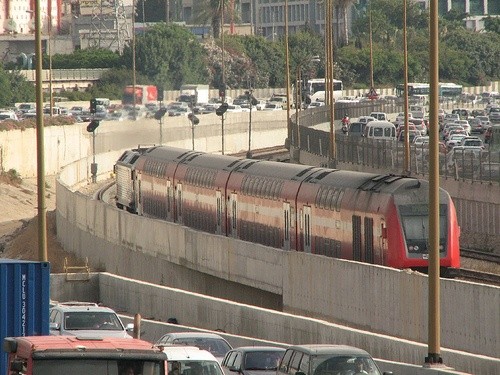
[113,146,460,273]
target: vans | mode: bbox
[358,115,376,124]
[397,112,412,121]
[44,105,61,116]
[462,135,484,167]
[348,121,367,135]
[408,104,425,118]
[443,113,460,123]
[96,97,110,108]
[369,110,390,122]
[361,120,397,149]
[94,104,105,116]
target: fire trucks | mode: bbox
[122,85,159,105]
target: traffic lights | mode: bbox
[303,75,308,91]
[91,98,96,113]
[219,82,225,96]
[158,86,163,100]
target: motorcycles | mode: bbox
[341,123,349,133]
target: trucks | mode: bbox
[271,93,293,110]
[179,84,210,104]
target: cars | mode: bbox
[220,346,286,374]
[20,112,36,119]
[111,110,122,119]
[264,103,282,110]
[80,109,93,121]
[449,134,467,140]
[158,331,233,365]
[397,129,421,145]
[396,122,416,138]
[337,95,358,103]
[70,105,83,113]
[163,345,225,375]
[446,140,462,146]
[447,129,467,136]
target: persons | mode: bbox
[346,358,374,374]
[94,313,115,329]
[271,353,282,368]
[341,114,351,127]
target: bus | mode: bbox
[291,79,345,103]
[395,82,463,98]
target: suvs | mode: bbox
[455,119,471,133]
[172,101,189,111]
[469,117,484,132]
[308,98,337,108]
[476,115,490,129]
[0,112,16,120]
[275,345,395,375]
[49,304,135,340]
[489,106,500,120]
[452,108,469,120]
[413,118,427,135]
[18,102,35,113]
